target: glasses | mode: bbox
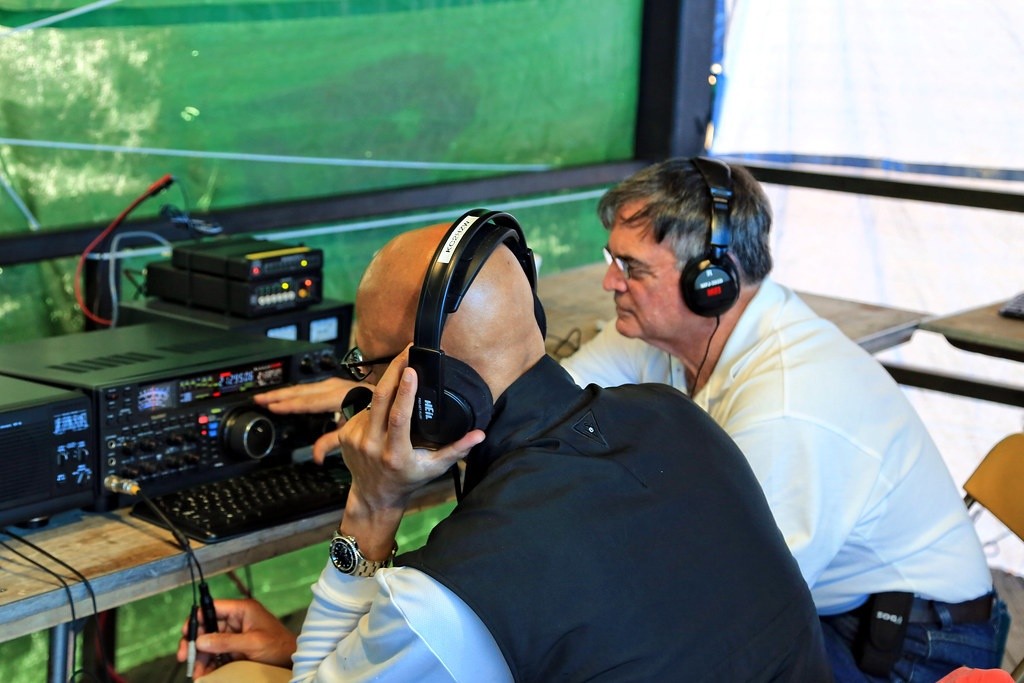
[339,346,397,382]
[600,245,660,280]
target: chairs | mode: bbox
[957,432,1024,683]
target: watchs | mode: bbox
[329,527,399,577]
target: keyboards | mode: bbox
[131,452,354,545]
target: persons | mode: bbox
[253,155,1011,683]
[174,221,829,682]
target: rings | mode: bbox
[367,402,372,410]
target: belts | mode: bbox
[849,594,996,624]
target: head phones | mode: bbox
[679,157,740,317]
[408,208,546,451]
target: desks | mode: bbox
[919,293,1024,362]
[0,259,932,683]
